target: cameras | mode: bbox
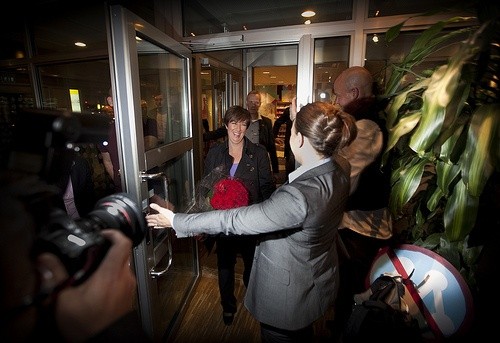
[34,192,149,284]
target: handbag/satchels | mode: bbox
[342,275,421,343]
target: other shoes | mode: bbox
[224,311,234,324]
[325,320,342,340]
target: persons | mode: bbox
[99,97,121,184]
[0,200,137,343]
[273,107,294,181]
[145,101,359,343]
[324,66,394,333]
[202,106,276,324]
[142,100,158,198]
[203,90,279,182]
